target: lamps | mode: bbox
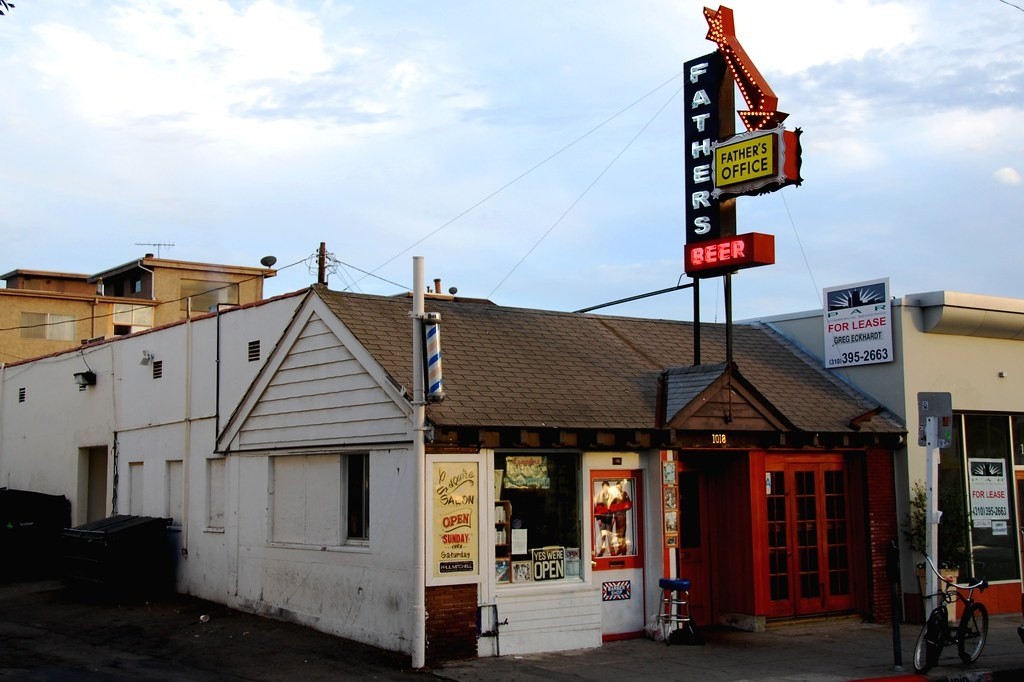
[73,372,96,386]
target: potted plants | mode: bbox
[902,482,974,621]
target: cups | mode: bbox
[200,615,209,622]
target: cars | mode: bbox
[973,523,1016,582]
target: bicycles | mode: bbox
[913,548,986,675]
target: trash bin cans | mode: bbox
[62,515,172,605]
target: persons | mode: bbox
[594,480,633,557]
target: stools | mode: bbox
[655,578,695,647]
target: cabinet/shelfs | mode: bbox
[495,501,512,584]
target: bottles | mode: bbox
[495,528,506,544]
